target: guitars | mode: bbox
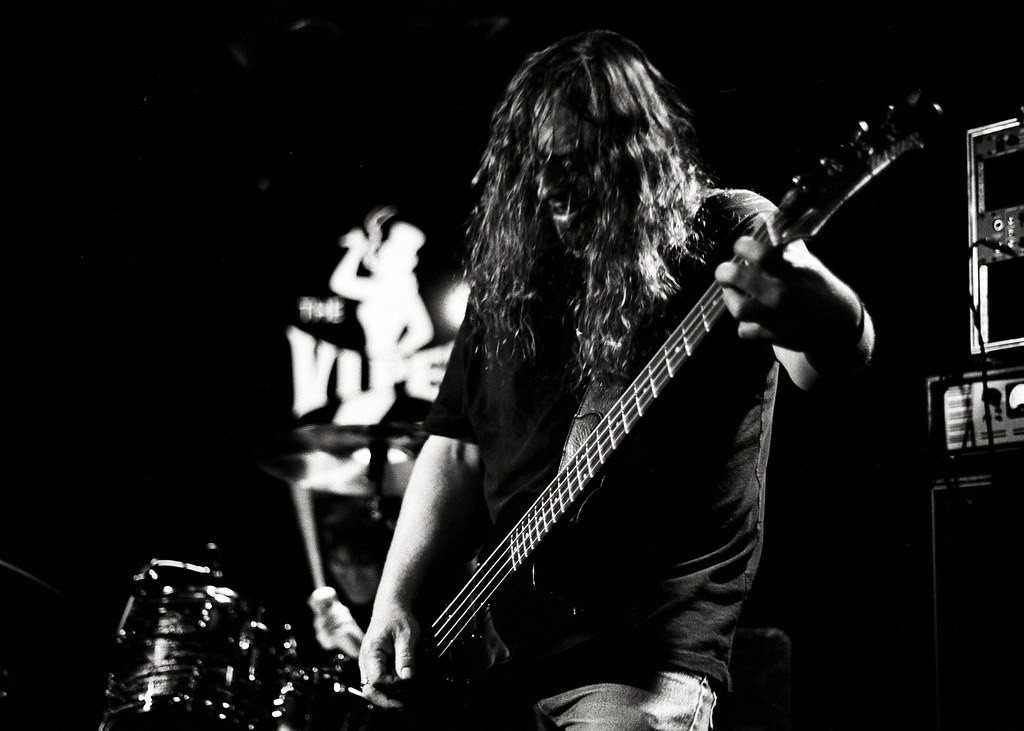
[393,81,950,728]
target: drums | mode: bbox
[102,583,268,724]
[259,662,404,729]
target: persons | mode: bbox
[242,200,438,614]
[357,28,875,731]
